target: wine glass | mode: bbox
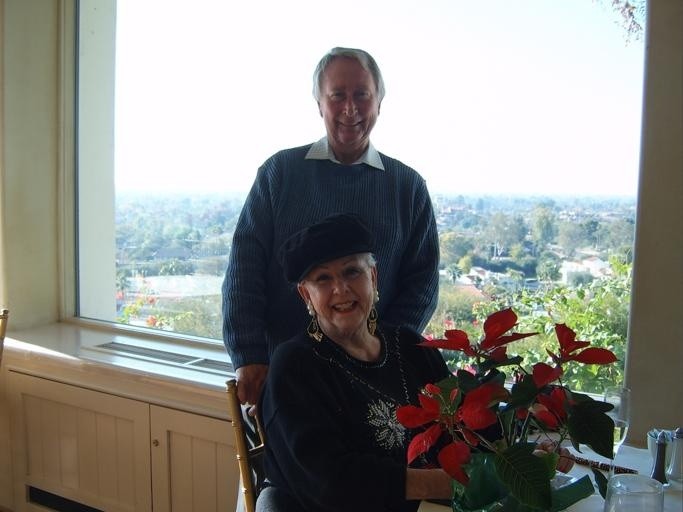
[593,386,633,489]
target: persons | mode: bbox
[258,213,576,512]
[220,45,440,511]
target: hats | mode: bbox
[277,212,375,281]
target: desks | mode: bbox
[416,440,682,511]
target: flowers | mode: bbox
[393,306,618,511]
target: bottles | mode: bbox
[666,436,683,479]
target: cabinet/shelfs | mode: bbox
[6,367,237,512]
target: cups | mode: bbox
[603,473,665,512]
[645,431,672,487]
